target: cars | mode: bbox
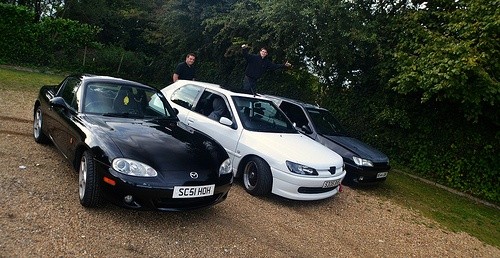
[33,72,234,213]
[255,93,390,187]
[148,79,347,203]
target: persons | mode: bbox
[241,43,292,93]
[173,52,197,83]
